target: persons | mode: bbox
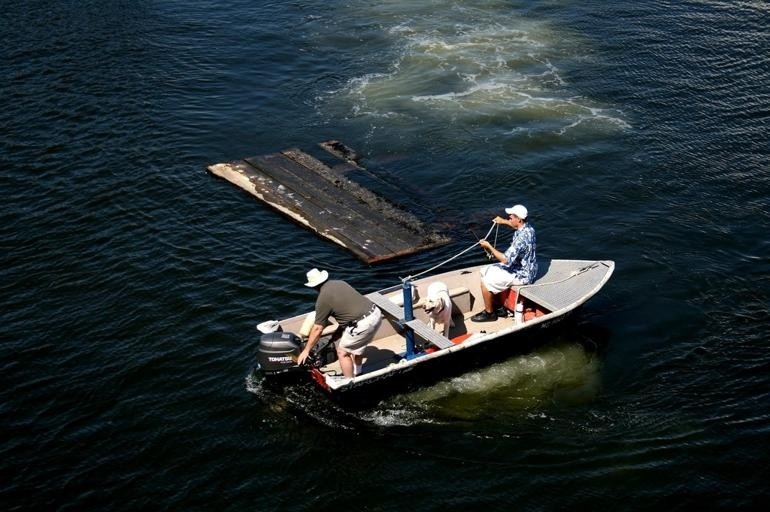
[469,203,538,322]
[296,268,382,380]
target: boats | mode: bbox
[257,255,626,404]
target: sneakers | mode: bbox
[471,309,498,322]
[494,304,508,317]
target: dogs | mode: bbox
[423,281,456,339]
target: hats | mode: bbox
[504,204,529,220]
[303,268,329,288]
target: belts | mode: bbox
[356,303,376,322]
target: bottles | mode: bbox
[515,304,523,322]
